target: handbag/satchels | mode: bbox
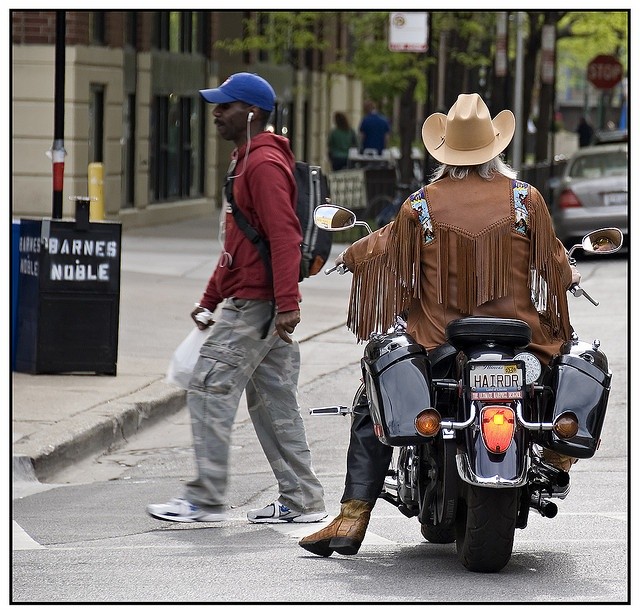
[228,162,331,339]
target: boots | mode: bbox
[543,448,577,472]
[298,500,371,557]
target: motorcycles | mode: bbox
[310,202,624,571]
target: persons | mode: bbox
[329,110,356,172]
[299,94,581,558]
[358,101,391,156]
[148,73,328,525]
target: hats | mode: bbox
[199,71,276,111]
[421,93,516,166]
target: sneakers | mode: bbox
[247,499,328,523]
[147,498,227,523]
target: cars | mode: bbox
[554,144,629,247]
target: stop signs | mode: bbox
[586,54,624,90]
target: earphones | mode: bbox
[217,110,256,269]
